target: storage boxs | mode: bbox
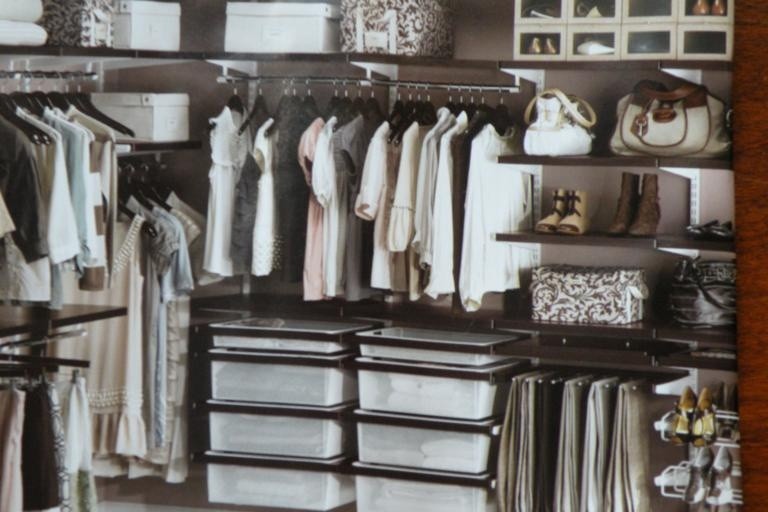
[114,0,182,51]
[37,0,114,47]
[531,265,643,325]
[224,2,341,53]
[341,0,456,58]
[91,90,190,142]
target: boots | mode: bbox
[607,171,639,237]
[532,187,570,236]
[627,171,663,236]
[556,189,591,235]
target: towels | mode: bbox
[0,0,49,47]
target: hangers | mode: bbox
[230,76,503,106]
[508,338,666,393]
[0,67,136,146]
[118,161,166,182]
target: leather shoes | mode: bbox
[710,220,736,242]
[683,216,719,240]
[668,385,745,508]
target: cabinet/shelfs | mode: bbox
[1,43,735,353]
[513,0,735,61]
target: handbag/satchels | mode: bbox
[663,257,735,328]
[606,76,734,163]
[518,86,596,158]
